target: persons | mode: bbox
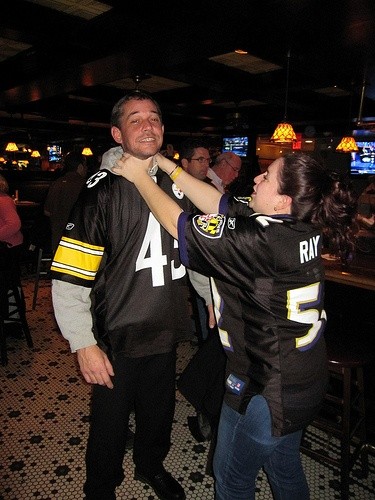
[44,152,89,256]
[0,175,24,271]
[50,90,217,499]
[158,142,241,218]
[112,154,358,499]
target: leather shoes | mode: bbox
[134,466,186,500]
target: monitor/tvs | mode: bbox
[351,141,375,175]
[223,137,248,157]
[49,145,61,162]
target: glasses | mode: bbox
[186,157,213,163]
[226,160,239,173]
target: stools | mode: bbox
[300,335,370,500]
[0,263,33,366]
[32,247,52,310]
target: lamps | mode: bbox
[269,49,297,145]
[335,81,359,154]
[81,144,93,155]
